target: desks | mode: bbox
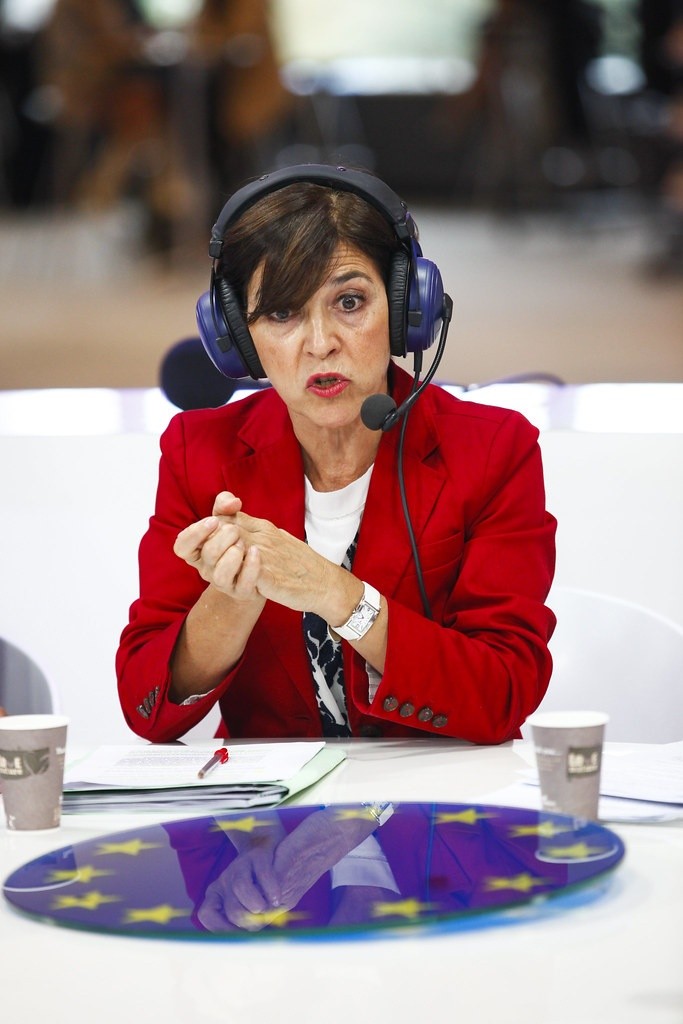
[0,736,683,1024]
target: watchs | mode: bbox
[361,801,395,827]
[330,581,382,641]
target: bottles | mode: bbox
[525,712,611,822]
[0,712,70,832]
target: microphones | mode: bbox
[361,294,453,432]
[159,336,276,412]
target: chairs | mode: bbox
[517,590,682,739]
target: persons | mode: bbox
[161,801,568,933]
[36,0,299,276]
[113,165,559,746]
[452,0,683,277]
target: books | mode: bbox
[60,739,346,816]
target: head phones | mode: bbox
[197,163,452,380]
